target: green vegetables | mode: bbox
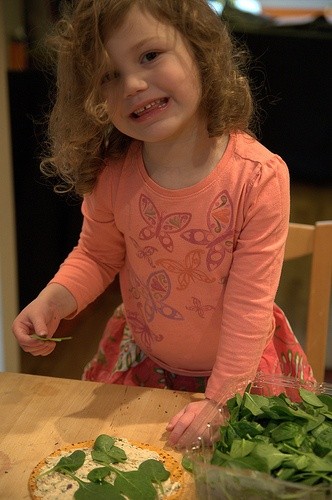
[29,335,72,342]
[207,383,332,500]
[28,435,184,500]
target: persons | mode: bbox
[9,0,317,450]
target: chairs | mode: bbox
[284,220,332,385]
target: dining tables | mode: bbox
[0,371,206,500]
